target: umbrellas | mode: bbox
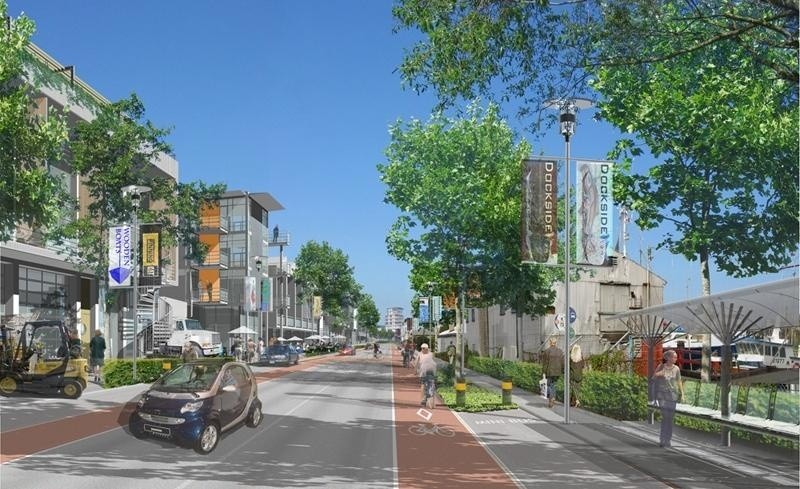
[305,334,347,343]
[288,335,303,341]
[277,336,286,341]
[228,326,260,335]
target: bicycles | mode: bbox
[366,350,385,361]
[418,373,440,408]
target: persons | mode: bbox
[56,326,82,359]
[373,338,383,358]
[446,339,456,365]
[649,349,684,450]
[410,342,438,406]
[182,340,199,363]
[246,337,257,363]
[205,279,213,302]
[398,337,417,367]
[198,280,205,303]
[569,344,585,409]
[257,337,265,357]
[272,224,280,243]
[267,336,276,349]
[90,329,106,382]
[541,337,564,408]
[233,335,244,362]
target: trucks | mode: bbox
[157,296,224,358]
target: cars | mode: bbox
[130,357,262,453]
[260,346,299,367]
[338,345,355,356]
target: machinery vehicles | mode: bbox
[3,320,88,400]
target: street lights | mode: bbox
[541,96,593,423]
